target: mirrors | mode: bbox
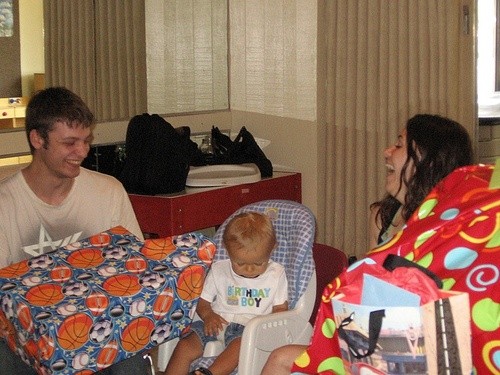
[0,0,230,185]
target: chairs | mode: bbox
[156,199,318,375]
[308,242,349,328]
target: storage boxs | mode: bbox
[0,225,217,375]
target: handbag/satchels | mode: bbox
[331,254,473,375]
[209,125,273,179]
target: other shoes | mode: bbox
[189,367,213,375]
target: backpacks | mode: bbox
[120,113,190,195]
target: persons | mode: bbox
[260,115,475,375]
[164,212,288,375]
[0,87,148,375]
